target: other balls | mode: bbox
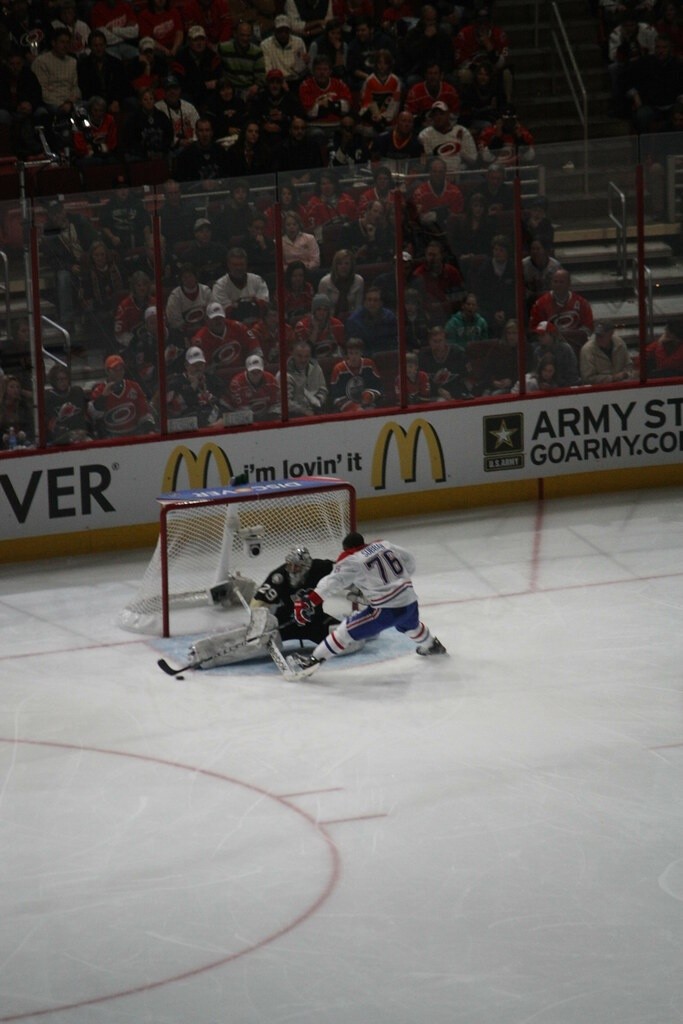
[176,676,184,680]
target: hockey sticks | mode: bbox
[157,619,296,676]
[233,586,321,682]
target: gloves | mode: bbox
[292,594,318,627]
[362,393,373,403]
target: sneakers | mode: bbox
[415,635,447,658]
[289,652,326,678]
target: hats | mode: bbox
[104,354,125,369]
[114,176,129,187]
[144,306,158,320]
[501,104,515,119]
[194,218,213,232]
[267,69,282,83]
[188,26,206,38]
[591,317,611,334]
[185,347,208,363]
[534,321,556,335]
[204,302,226,319]
[138,37,154,52]
[245,354,264,372]
[273,15,292,30]
[428,101,449,117]
[310,295,331,320]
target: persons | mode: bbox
[286,532,449,675]
[188,544,365,669]
[0,0,683,445]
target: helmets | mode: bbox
[285,546,311,577]
